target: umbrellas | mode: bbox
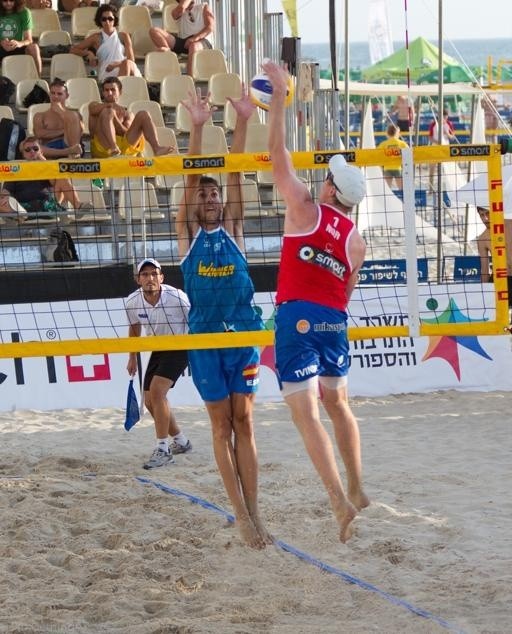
[453,165,512,219]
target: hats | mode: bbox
[137,258,161,271]
[329,154,366,207]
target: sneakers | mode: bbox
[170,440,192,454]
[144,447,174,469]
[75,202,93,219]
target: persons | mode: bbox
[261,60,370,543]
[176,82,274,548]
[125,258,191,469]
[377,94,456,195]
[477,206,512,309]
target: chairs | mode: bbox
[0,0,317,226]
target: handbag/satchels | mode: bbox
[41,45,69,58]
[25,85,50,108]
[43,230,79,262]
[0,117,26,160]
[1,76,16,106]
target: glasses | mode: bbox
[102,16,113,22]
[326,172,343,195]
[189,12,194,22]
[25,146,39,152]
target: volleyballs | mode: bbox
[248,72,295,112]
